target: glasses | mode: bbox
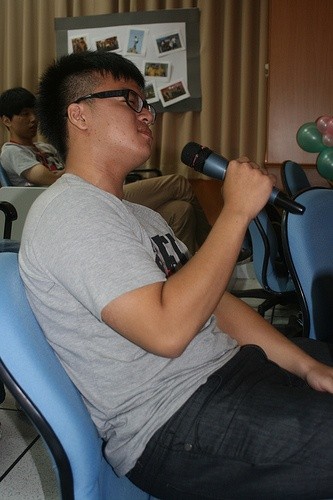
[64,88,156,127]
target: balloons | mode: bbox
[297,116,333,181]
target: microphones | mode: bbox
[181,142,305,214]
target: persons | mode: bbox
[0,87,211,256]
[73,36,184,102]
[17,51,333,500]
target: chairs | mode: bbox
[281,187,333,340]
[230,207,297,318]
[0,239,158,500]
[281,160,311,198]
